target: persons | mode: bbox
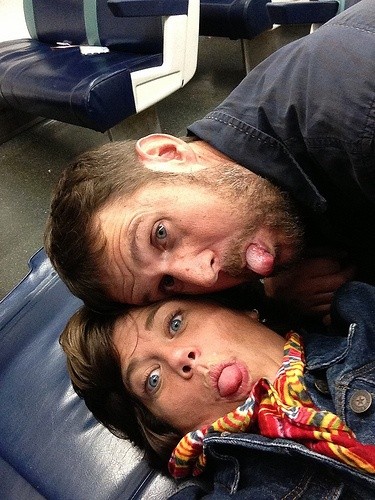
[58,281,375,500]
[44,0,375,319]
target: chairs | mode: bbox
[0,0,340,143]
[0,242,200,499]
